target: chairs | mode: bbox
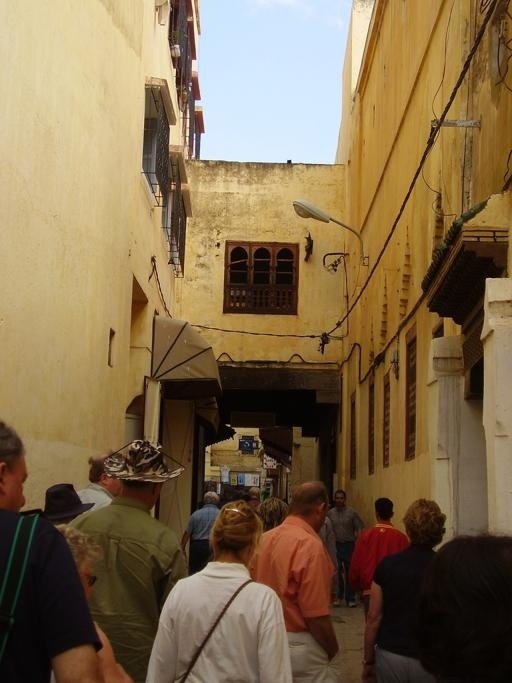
[333,601,341,607]
[348,600,356,607]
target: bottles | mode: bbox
[103,439,185,485]
[43,484,96,521]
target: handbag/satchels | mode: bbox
[360,657,375,666]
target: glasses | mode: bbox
[291,198,369,266]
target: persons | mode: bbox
[39,479,96,531]
[143,499,294,682]
[412,533,510,682]
[52,521,137,683]
[346,494,410,625]
[72,446,123,511]
[1,418,105,682]
[180,483,364,610]
[244,478,342,682]
[63,438,191,683]
[358,499,448,680]
